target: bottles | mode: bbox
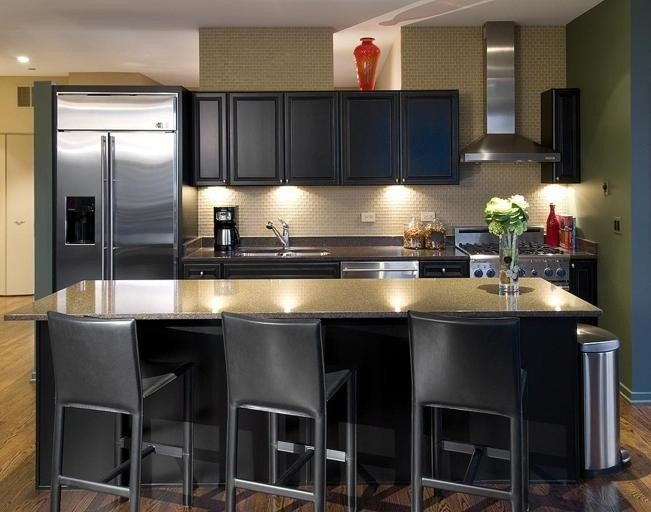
[425,217,447,250]
[403,216,425,249]
[546,203,559,248]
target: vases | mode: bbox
[497,231,521,297]
[353,37,381,91]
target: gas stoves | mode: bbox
[456,242,570,282]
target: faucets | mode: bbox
[266,217,289,247]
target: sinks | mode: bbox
[239,248,331,257]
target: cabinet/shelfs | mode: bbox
[541,88,581,183]
[421,260,468,277]
[343,91,459,185]
[183,263,220,279]
[186,90,229,185]
[230,90,342,186]
[570,259,598,326]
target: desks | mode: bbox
[3,279,603,489]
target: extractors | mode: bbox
[459,21,561,163]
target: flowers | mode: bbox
[484,195,530,237]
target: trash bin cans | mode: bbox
[576,323,631,475]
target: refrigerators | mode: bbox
[56,93,178,292]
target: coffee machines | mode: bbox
[213,207,239,251]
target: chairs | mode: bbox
[220,309,364,512]
[46,310,197,512]
[404,308,532,512]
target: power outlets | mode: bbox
[360,212,376,223]
